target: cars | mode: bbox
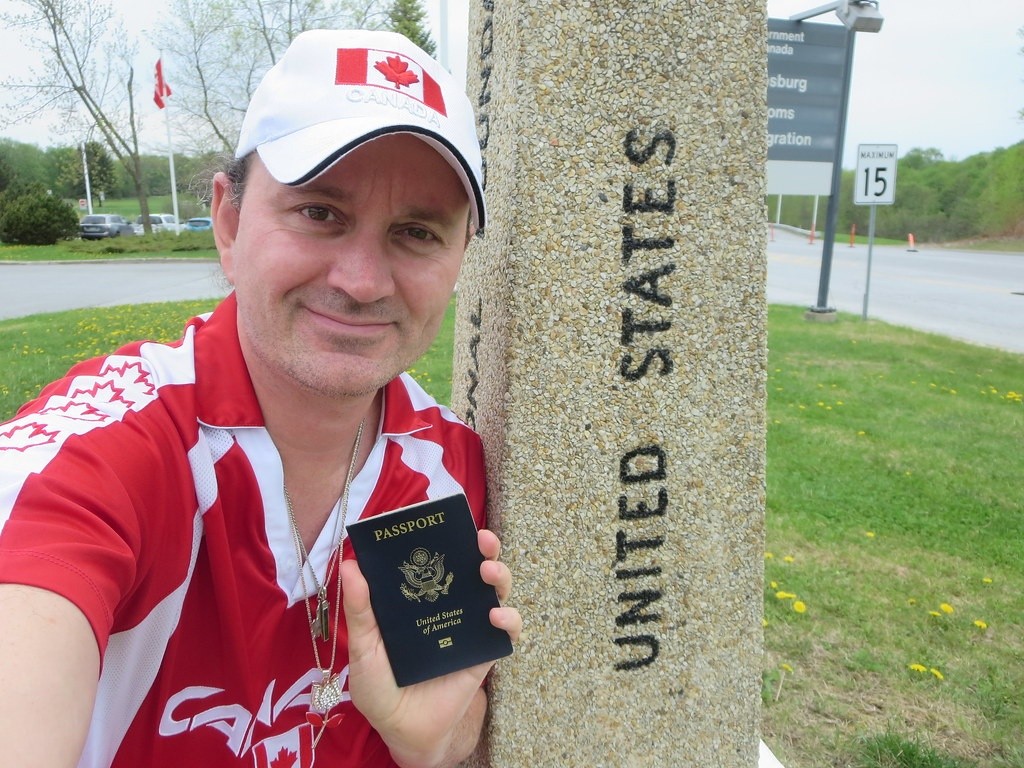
[79,214,134,241]
[134,214,186,235]
[186,218,212,232]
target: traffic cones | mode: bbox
[770,224,775,242]
[809,223,815,244]
[907,233,918,252]
[848,224,855,248]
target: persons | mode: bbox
[0,28,522,768]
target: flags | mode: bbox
[153,59,171,108]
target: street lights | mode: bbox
[789,0,884,322]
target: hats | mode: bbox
[235,29,487,229]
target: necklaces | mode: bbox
[285,429,362,640]
[283,418,364,749]
